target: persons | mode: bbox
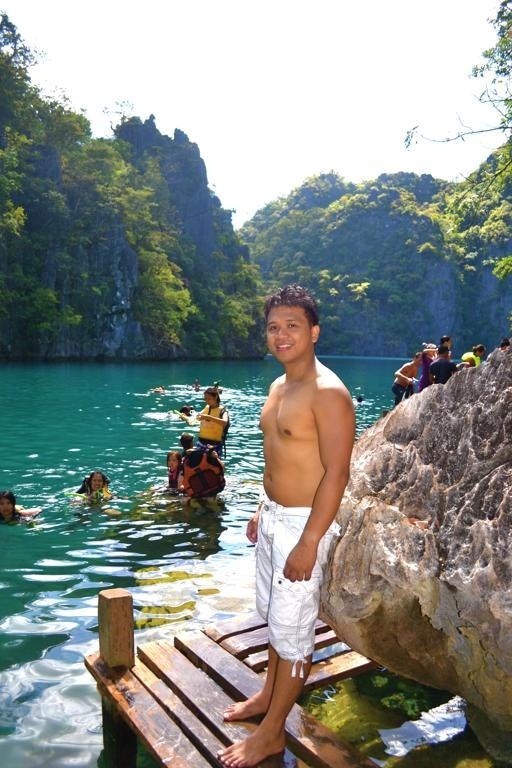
[152,384,170,392]
[191,376,202,391]
[417,340,439,392]
[427,345,461,385]
[392,350,424,406]
[213,381,222,394]
[462,343,487,370]
[357,394,364,402]
[213,280,356,767]
[500,336,511,350]
[440,336,455,363]
[178,387,231,458]
[165,404,201,426]
[0,487,45,527]
[120,448,184,502]
[176,432,227,515]
[58,468,124,522]
